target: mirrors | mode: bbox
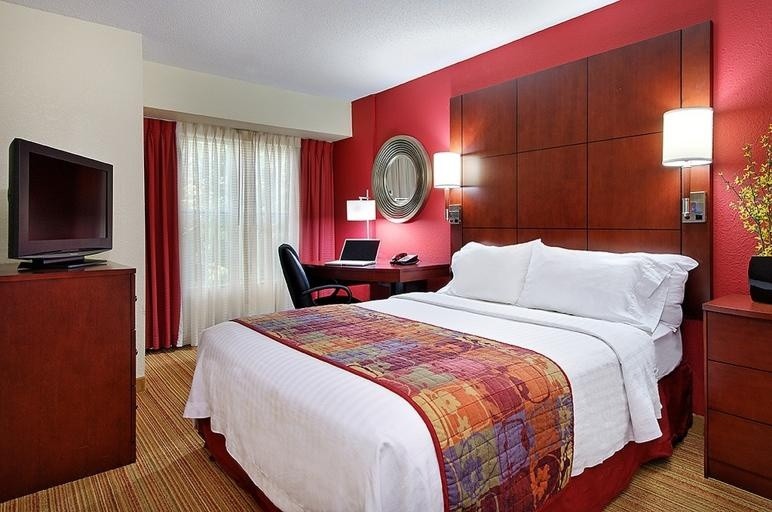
[371,135,433,224]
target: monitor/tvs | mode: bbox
[7,137,114,269]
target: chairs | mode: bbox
[277,244,364,309]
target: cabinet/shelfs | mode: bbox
[0,259,138,503]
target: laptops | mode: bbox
[325,239,381,266]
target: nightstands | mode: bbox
[703,293,772,500]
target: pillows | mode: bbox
[450,238,698,333]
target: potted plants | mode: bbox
[718,123,772,304]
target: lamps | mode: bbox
[433,151,462,224]
[346,189,377,239]
[661,107,714,224]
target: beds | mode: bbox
[196,20,713,512]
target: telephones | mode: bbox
[390,253,418,263]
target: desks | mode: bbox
[301,258,450,296]
